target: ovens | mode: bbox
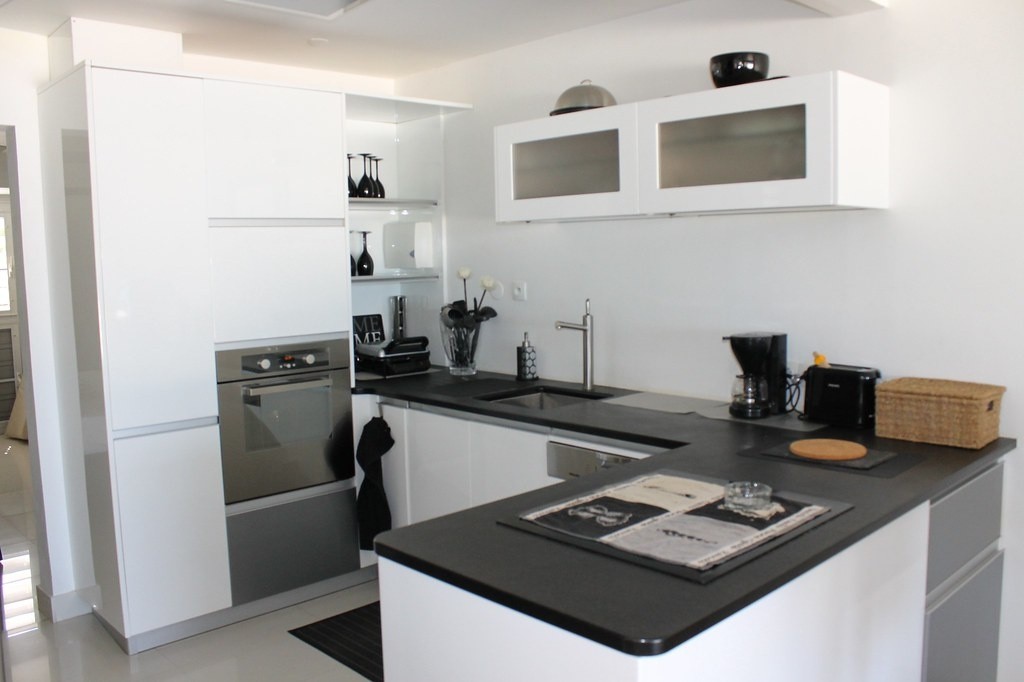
[211,337,357,609]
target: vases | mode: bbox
[438,315,481,376]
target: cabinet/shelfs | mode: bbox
[491,70,894,226]
[349,194,448,280]
[377,401,409,526]
[202,74,353,346]
[38,59,235,642]
[409,409,547,525]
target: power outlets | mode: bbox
[511,282,527,302]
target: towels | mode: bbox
[356,417,396,551]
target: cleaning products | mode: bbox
[516,332,538,381]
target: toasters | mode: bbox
[801,364,878,428]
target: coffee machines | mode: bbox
[724,333,792,419]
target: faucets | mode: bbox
[554,299,594,391]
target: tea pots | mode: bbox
[732,373,770,407]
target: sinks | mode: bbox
[473,385,614,411]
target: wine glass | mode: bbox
[355,231,374,276]
[346,153,387,199]
[350,231,356,276]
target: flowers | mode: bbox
[442,268,499,364]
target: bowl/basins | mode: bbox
[708,51,771,88]
